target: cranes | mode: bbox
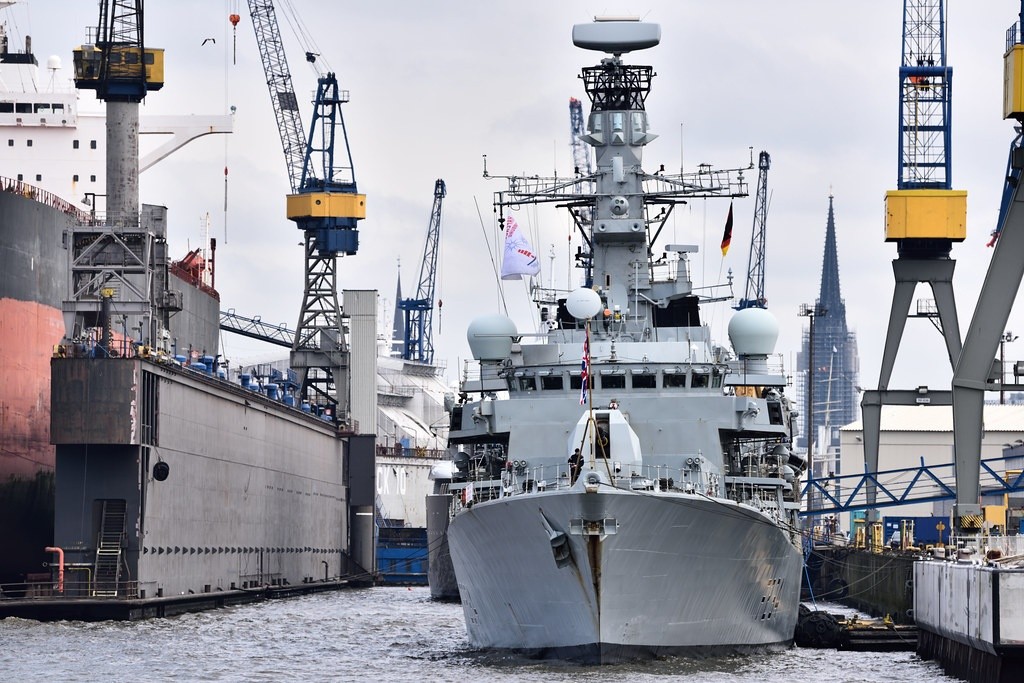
[245,0,367,418]
[882,0,968,241]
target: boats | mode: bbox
[445,13,805,667]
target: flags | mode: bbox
[500,210,542,281]
[720,202,733,256]
[579,328,589,406]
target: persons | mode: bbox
[825,516,836,534]
[567,449,584,486]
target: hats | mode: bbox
[575,449,579,452]
